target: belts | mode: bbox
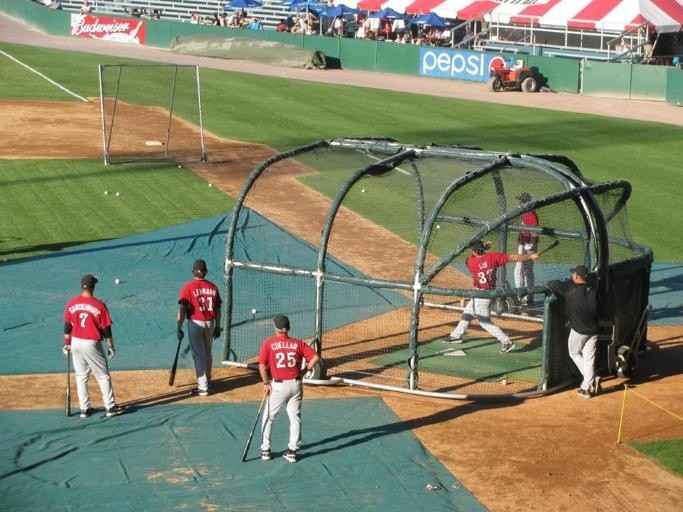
[275,376,301,382]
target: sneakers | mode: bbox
[578,389,591,398]
[259,450,271,460]
[80,409,95,417]
[281,451,297,462]
[442,336,463,343]
[592,375,601,394]
[105,406,124,417]
[192,382,211,396]
[499,340,515,353]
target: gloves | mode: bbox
[62,344,71,355]
[177,330,184,339]
[108,345,115,359]
[213,327,220,340]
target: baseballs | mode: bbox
[209,183,212,188]
[437,225,440,229]
[502,380,507,385]
[104,191,108,195]
[116,192,120,196]
[115,279,120,284]
[361,188,367,193]
[251,308,256,314]
[178,165,182,169]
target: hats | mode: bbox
[274,316,289,330]
[570,266,588,277]
[470,240,484,249]
[515,193,531,202]
[81,274,98,289]
[194,260,206,272]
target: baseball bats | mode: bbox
[242,389,268,460]
[538,241,559,254]
[66,350,72,418]
[169,339,181,386]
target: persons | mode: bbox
[635,25,683,56]
[79,0,91,15]
[173,258,222,395]
[547,266,604,398]
[442,240,540,352]
[511,192,538,308]
[186,1,454,48]
[256,313,320,463]
[63,274,126,419]
[49,0,62,10]
[119,5,160,21]
